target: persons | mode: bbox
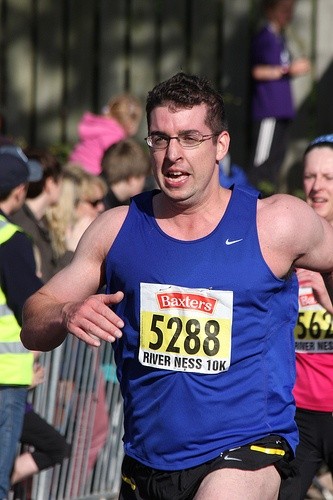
[0,0,332,499]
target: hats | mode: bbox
[0,141,43,192]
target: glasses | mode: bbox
[144,130,219,149]
